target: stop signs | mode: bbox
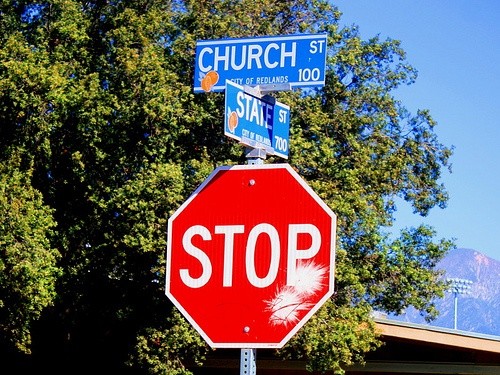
[165,164,336,348]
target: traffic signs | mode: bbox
[194,33,327,93]
[223,79,290,159]
